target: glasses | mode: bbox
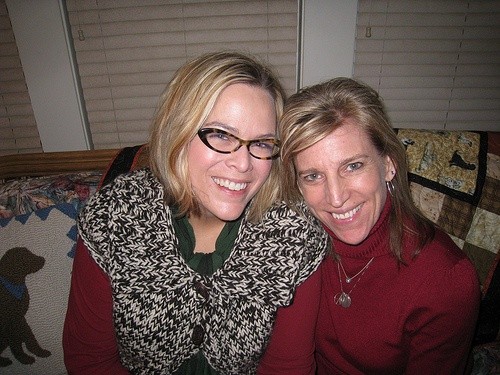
[197,128,283,162]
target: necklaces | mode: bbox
[334,251,375,308]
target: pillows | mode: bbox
[0,197,82,375]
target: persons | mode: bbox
[254,77,482,375]
[63,50,328,375]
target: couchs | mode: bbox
[1,126,500,375]
